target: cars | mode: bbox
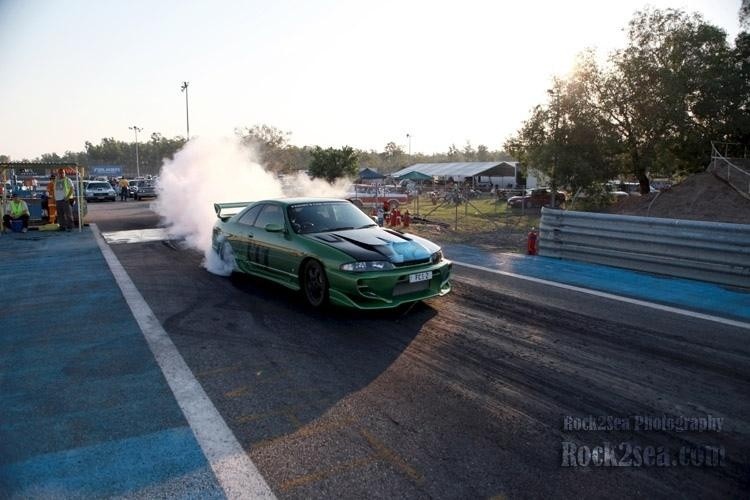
[508,188,566,209]
[3,176,160,202]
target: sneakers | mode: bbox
[22,227,27,233]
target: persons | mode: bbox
[54,168,74,232]
[31,175,37,192]
[46,174,58,224]
[3,194,30,233]
[109,175,130,201]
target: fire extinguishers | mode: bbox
[370,206,411,228]
[528,227,538,255]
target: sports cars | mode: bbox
[212,197,453,311]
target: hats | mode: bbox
[50,174,57,179]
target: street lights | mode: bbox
[129,126,144,175]
[181,81,191,143]
